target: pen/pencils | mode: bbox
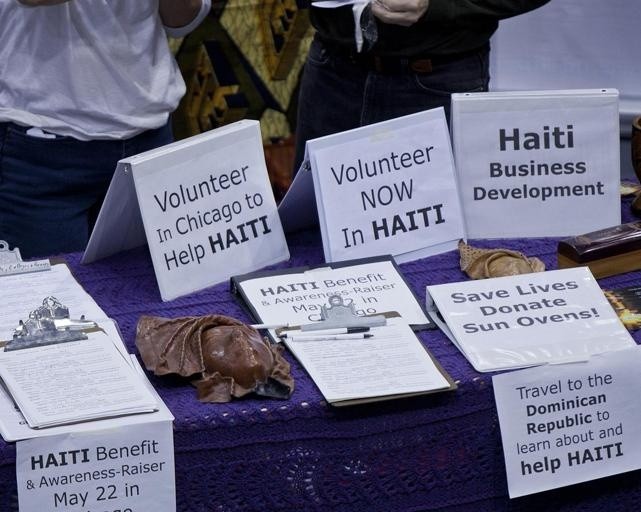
[279,327,376,341]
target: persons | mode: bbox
[0,1,214,260]
[287,1,552,185]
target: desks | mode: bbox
[1,228,639,512]
